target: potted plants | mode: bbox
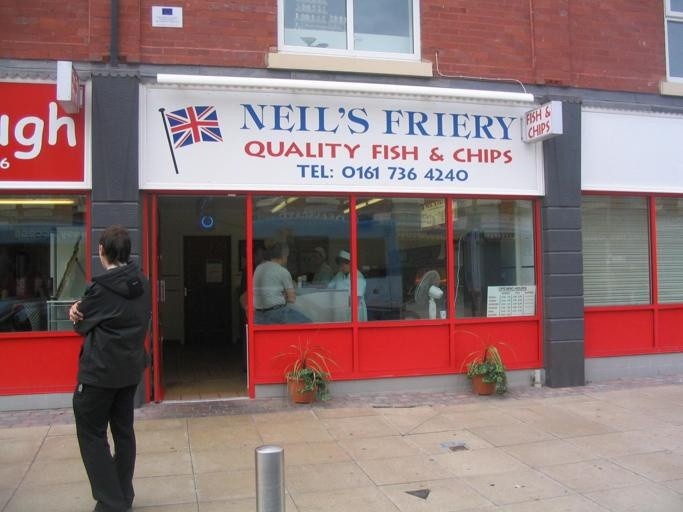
[452,330,512,396]
[273,336,338,408]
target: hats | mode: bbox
[340,250,351,261]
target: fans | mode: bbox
[415,270,444,320]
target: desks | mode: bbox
[47,298,77,332]
[287,288,349,322]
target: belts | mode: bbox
[256,301,288,313]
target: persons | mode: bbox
[165,103,224,152]
[326,250,369,323]
[67,224,154,511]
[251,241,314,324]
[304,244,332,282]
